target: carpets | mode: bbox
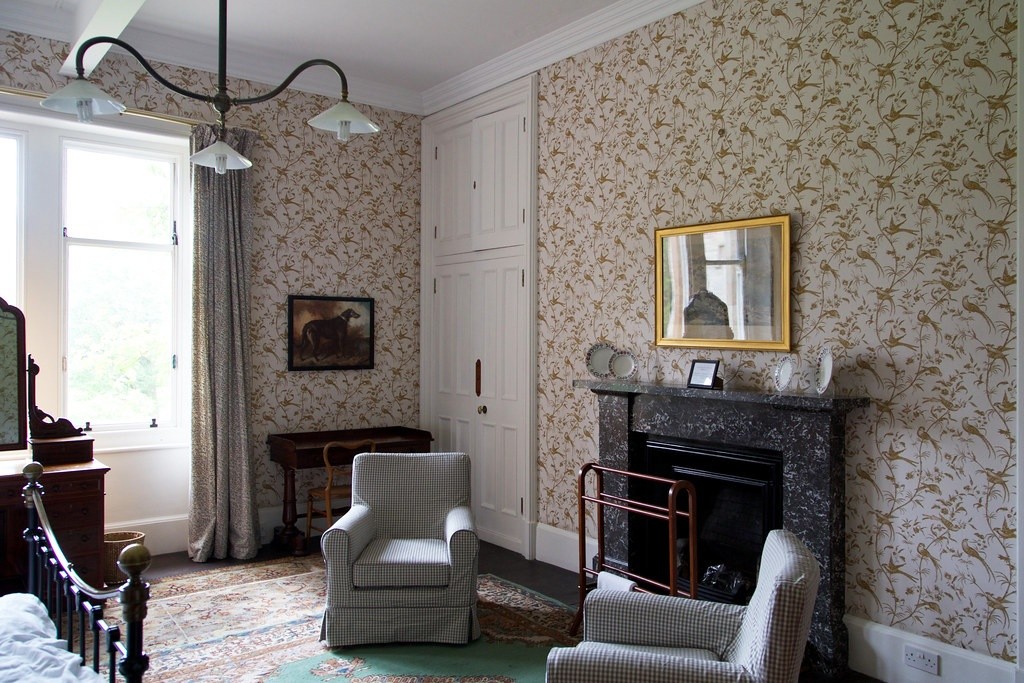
[53,553,581,683]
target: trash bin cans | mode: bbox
[104,530,146,590]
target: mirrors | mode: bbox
[654,212,791,351]
[0,297,26,451]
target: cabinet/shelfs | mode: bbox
[1,457,111,633]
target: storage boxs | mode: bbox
[27,436,93,464]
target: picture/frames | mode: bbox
[686,359,720,391]
[289,295,375,371]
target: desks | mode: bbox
[268,426,435,556]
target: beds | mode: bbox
[1,461,151,683]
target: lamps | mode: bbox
[39,1,380,175]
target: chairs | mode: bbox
[305,440,376,552]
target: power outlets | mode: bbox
[905,644,940,676]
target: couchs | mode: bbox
[318,451,480,650]
[545,529,821,683]
